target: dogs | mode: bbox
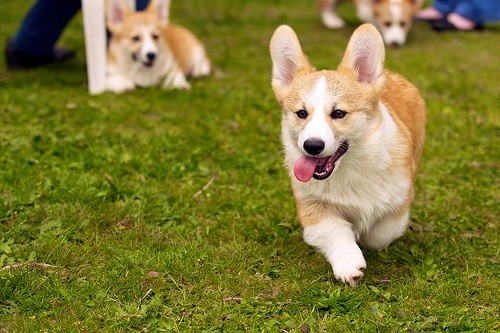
[316,0,424,49]
[269,22,427,288]
[105,0,212,96]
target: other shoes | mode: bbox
[411,13,446,24]
[432,18,484,33]
[4,44,75,69]
